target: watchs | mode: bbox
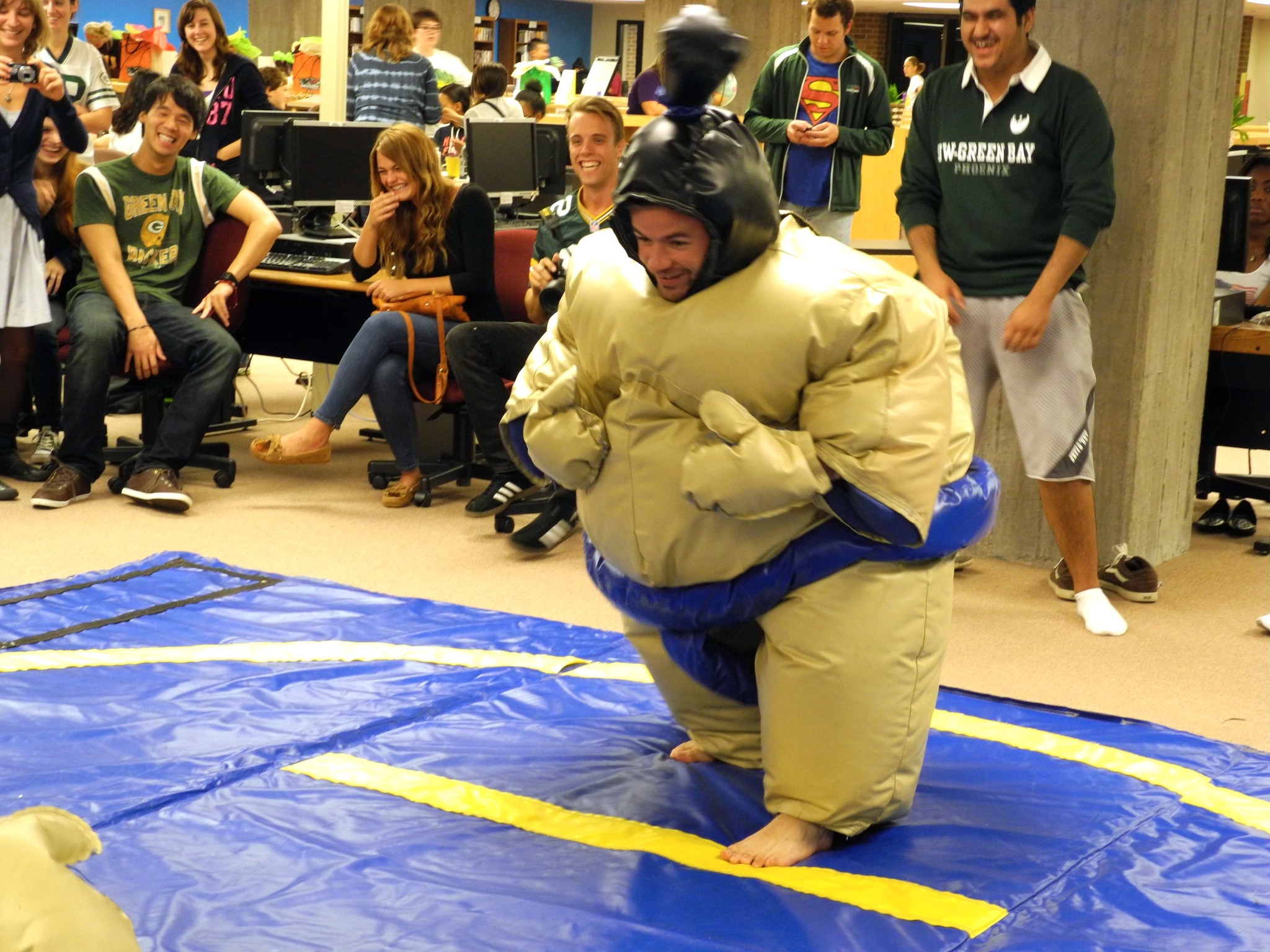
[221,273,238,285]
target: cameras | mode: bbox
[5,62,39,84]
[539,262,567,318]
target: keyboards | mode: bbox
[256,251,352,275]
[495,219,541,231]
[1245,304,1270,320]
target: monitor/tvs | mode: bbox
[239,110,393,241]
[1216,176,1253,272]
[463,116,567,220]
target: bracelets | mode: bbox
[214,280,238,309]
[129,324,149,332]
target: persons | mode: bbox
[895,0,1128,635]
[626,50,668,116]
[444,92,625,550]
[1214,150,1270,307]
[511,38,622,96]
[0,0,291,515]
[345,3,472,139]
[747,0,894,245]
[500,14,1006,867]
[249,123,498,506]
[904,55,925,127]
[433,61,546,173]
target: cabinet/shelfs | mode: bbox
[472,16,495,71]
[347,4,365,63]
[496,18,548,86]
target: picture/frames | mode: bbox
[153,8,171,34]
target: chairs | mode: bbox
[363,225,539,510]
[97,208,250,497]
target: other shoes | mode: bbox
[0,452,48,482]
[251,434,332,466]
[382,472,425,507]
[0,481,20,501]
[1229,499,1257,536]
[1196,498,1231,535]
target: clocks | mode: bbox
[485,0,502,22]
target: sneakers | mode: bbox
[1048,556,1076,601]
[31,456,91,508]
[31,426,59,463]
[1095,542,1159,604]
[120,466,192,512]
[510,490,581,555]
[464,469,541,518]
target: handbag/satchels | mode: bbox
[371,292,470,323]
[118,32,164,84]
[291,44,322,96]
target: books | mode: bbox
[473,26,493,63]
[518,29,546,54]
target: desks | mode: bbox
[211,248,410,364]
[1193,306,1270,506]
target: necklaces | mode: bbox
[1250,246,1264,261]
[4,84,15,101]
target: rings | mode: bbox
[529,267,534,271]
